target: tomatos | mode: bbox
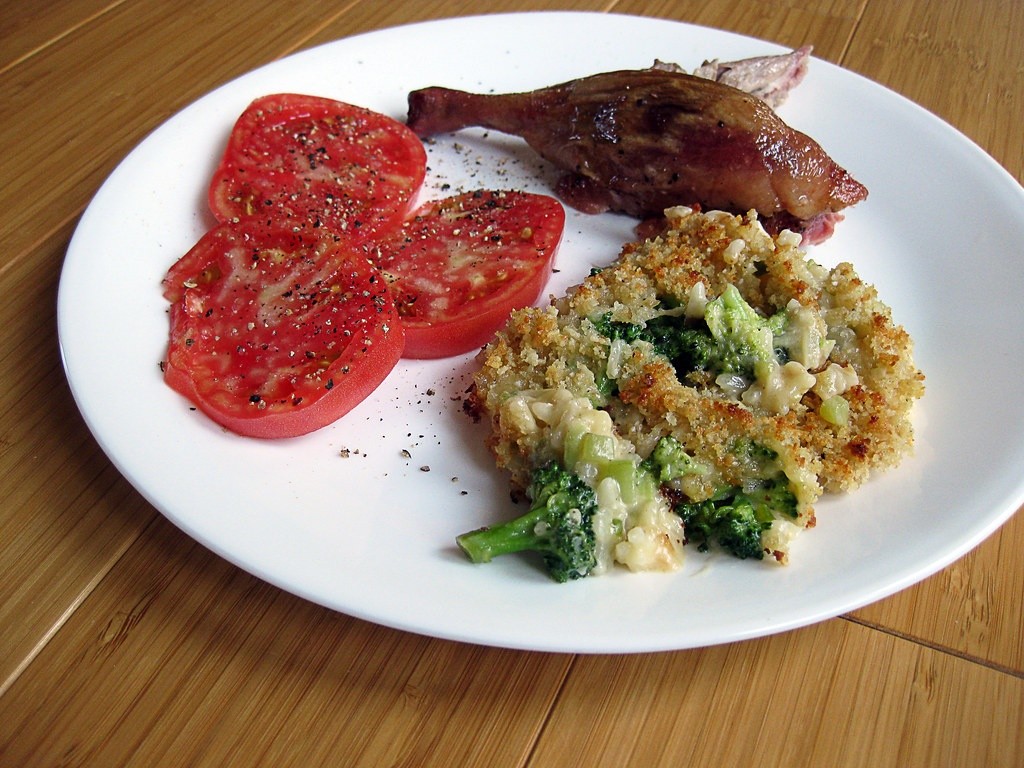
[162,93,566,439]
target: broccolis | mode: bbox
[451,290,807,583]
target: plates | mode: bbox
[55,11,1023,655]
